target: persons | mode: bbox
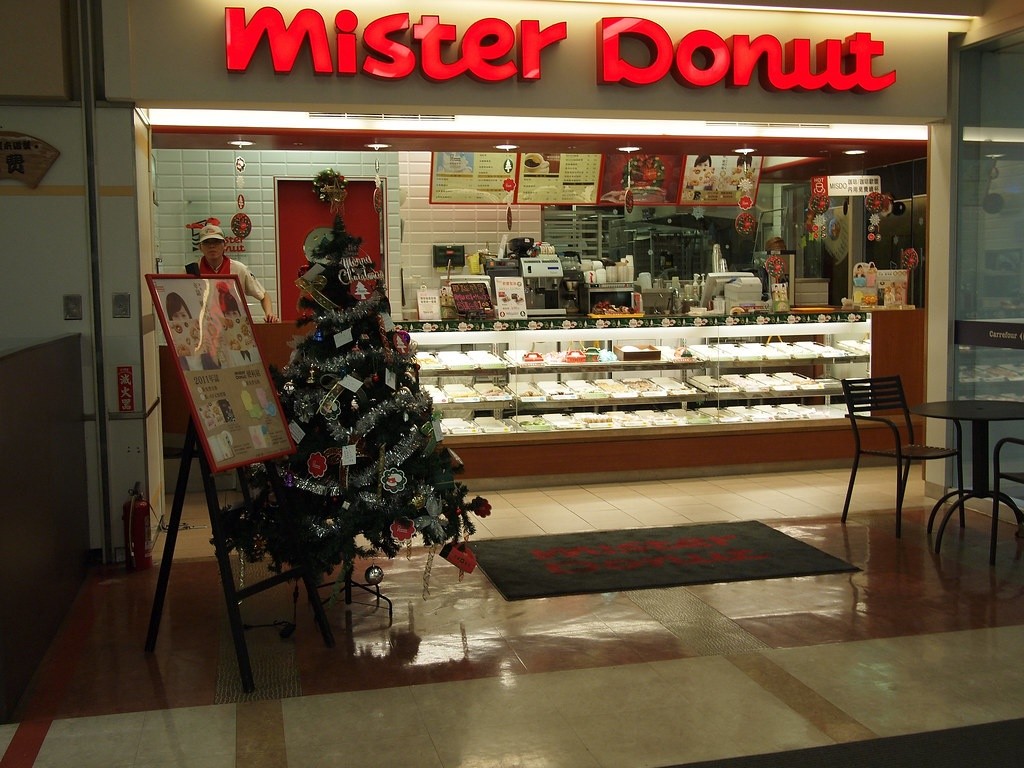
[218,291,261,366]
[772,285,780,298]
[166,292,220,371]
[854,265,865,278]
[186,225,279,323]
[684,155,719,191]
[781,284,786,300]
[737,155,752,170]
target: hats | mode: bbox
[199,225,225,243]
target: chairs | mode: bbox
[991,437,1024,539]
[841,374,966,538]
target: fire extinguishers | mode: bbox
[124,482,154,574]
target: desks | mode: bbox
[909,400,1024,554]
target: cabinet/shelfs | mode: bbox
[544,210,603,260]
[395,308,926,484]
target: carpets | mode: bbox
[469,521,865,602]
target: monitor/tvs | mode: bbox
[439,275,491,310]
[699,272,755,305]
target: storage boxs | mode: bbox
[614,345,661,361]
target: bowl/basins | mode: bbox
[523,153,544,172]
[584,266,628,283]
